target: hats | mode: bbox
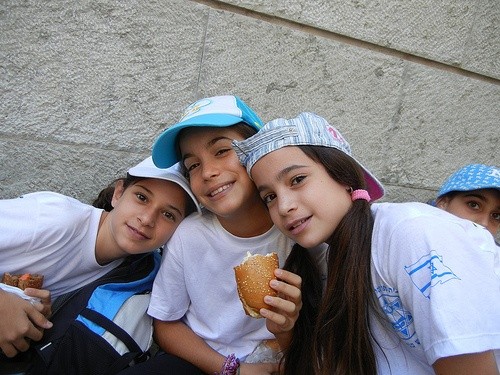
[231,111,385,202]
[436,163,500,198]
[128,155,206,215]
[152,95,265,169]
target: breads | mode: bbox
[1,272,45,290]
[233,251,279,317]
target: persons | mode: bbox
[146,93,329,375]
[232,110,500,375]
[0,155,203,357]
[434,164,500,234]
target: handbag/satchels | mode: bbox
[0,251,170,375]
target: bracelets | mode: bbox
[219,353,240,375]
